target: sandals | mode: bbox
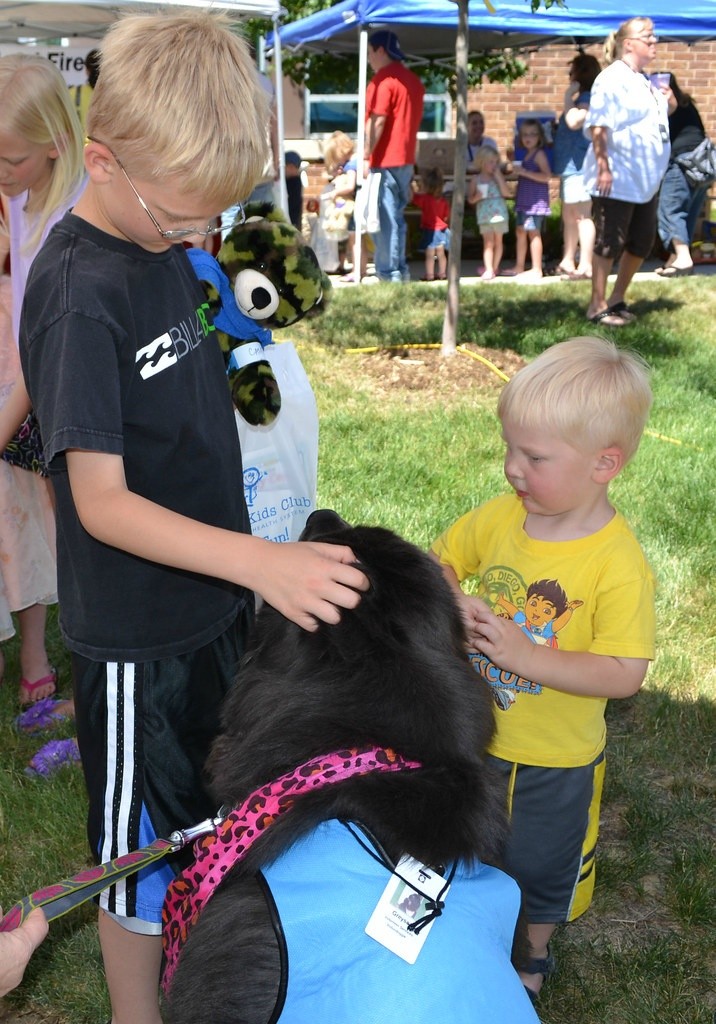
[565,270,592,279]
[418,273,434,281]
[543,266,575,276]
[433,272,446,280]
[510,943,552,1009]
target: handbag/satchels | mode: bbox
[668,137,715,188]
[354,172,381,232]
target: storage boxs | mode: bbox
[416,139,456,173]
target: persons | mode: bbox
[0,905,48,998]
[246,15,710,326]
[19,13,370,1024]
[0,50,100,773]
[426,337,657,1005]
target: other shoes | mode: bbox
[498,269,519,276]
[339,274,354,282]
[514,272,542,283]
[477,266,500,275]
[481,271,495,280]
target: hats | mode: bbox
[369,30,404,60]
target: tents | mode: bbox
[0,0,290,218]
[256,0,716,282]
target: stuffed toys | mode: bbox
[184,201,333,428]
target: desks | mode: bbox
[411,174,520,180]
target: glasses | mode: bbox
[623,33,659,42]
[568,71,575,77]
[86,135,245,241]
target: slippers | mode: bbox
[607,302,637,321]
[588,309,632,326]
[18,666,57,709]
[656,265,676,275]
[659,265,693,277]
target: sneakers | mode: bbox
[361,275,402,285]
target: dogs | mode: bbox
[155,510,540,1024]
